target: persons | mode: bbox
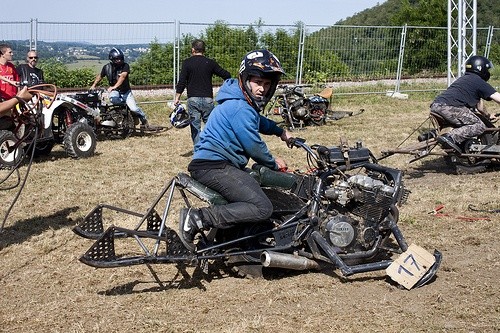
[179,49,297,251]
[0,44,21,101]
[430,55,500,156]
[174,39,231,142]
[16,51,44,87]
[0,85,35,118]
[89,48,149,129]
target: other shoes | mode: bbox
[418,134,430,150]
[435,134,462,156]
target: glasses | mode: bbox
[29,57,38,59]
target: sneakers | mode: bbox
[178,207,209,251]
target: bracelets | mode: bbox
[15,96,22,102]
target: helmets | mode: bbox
[169,103,195,129]
[238,48,288,112]
[108,47,125,67]
[464,55,494,81]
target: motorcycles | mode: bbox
[0,93,96,170]
[265,85,334,132]
[65,90,134,141]
[378,112,500,175]
[72,135,412,279]
